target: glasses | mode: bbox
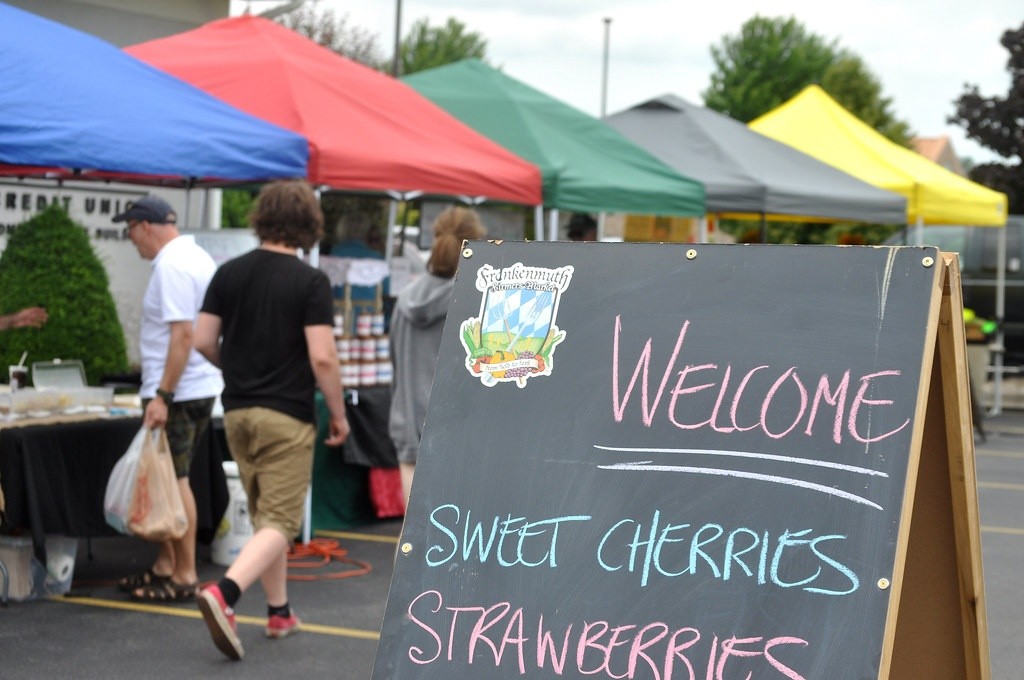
[124,221,143,237]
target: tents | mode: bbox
[1,11,546,550]
[207,54,708,267]
[595,93,908,248]
[706,82,1008,419]
[1,2,311,262]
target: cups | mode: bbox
[7,364,29,392]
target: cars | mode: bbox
[883,216,1024,378]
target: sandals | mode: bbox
[128,578,197,602]
[118,568,172,590]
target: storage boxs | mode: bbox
[206,460,255,567]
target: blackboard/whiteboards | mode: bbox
[369,237,995,680]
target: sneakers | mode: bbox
[197,582,244,660]
[266,609,300,638]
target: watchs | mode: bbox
[155,389,175,404]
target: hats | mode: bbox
[111,197,177,226]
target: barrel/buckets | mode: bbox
[210,460,259,567]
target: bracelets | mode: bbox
[327,412,347,420]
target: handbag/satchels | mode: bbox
[104,422,161,535]
[128,421,190,540]
[209,484,258,566]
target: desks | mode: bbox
[0,385,226,597]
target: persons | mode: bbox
[190,176,352,660]
[388,205,489,515]
[1,305,50,332]
[558,212,599,243]
[112,196,224,604]
